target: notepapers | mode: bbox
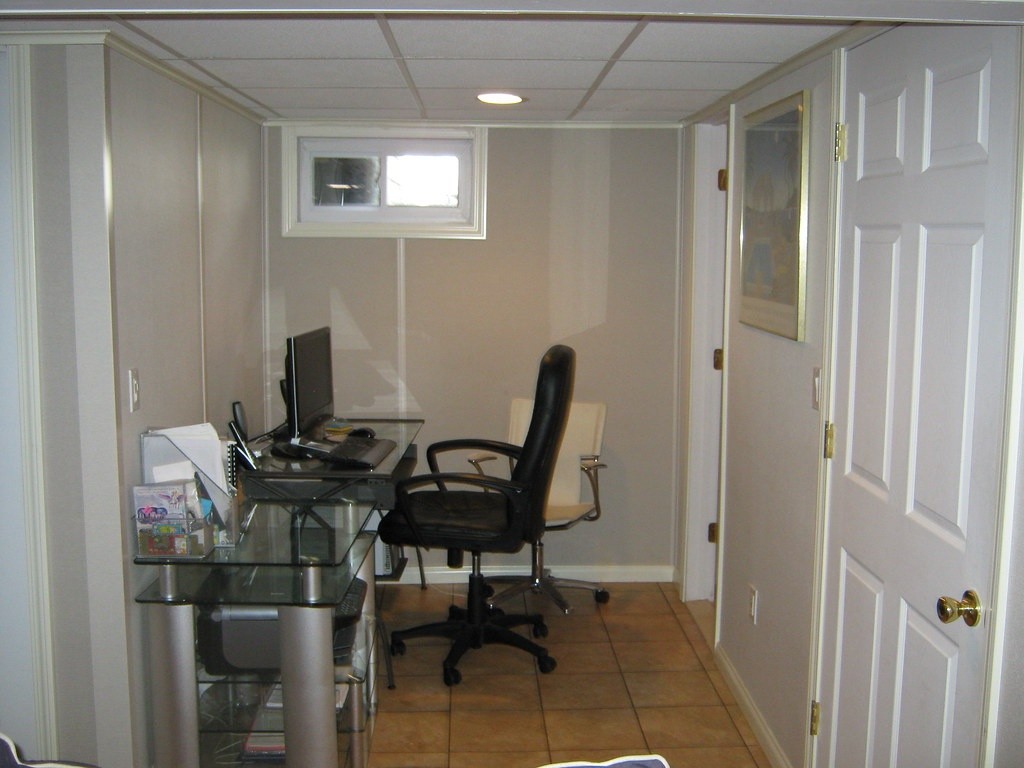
[325,423,353,432]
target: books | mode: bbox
[133,478,203,556]
[242,674,351,759]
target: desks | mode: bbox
[132,417,425,768]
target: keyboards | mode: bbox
[324,436,397,469]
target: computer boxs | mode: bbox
[293,503,401,576]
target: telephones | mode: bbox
[228,421,259,471]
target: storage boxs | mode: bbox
[136,525,215,559]
[133,482,188,555]
[166,479,205,523]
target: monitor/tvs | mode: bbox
[270,327,337,459]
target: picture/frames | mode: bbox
[736,89,812,344]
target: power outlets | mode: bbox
[744,583,758,625]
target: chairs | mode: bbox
[376,344,576,687]
[467,397,611,616]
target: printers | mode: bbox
[196,565,368,677]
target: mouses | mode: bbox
[349,426,379,438]
